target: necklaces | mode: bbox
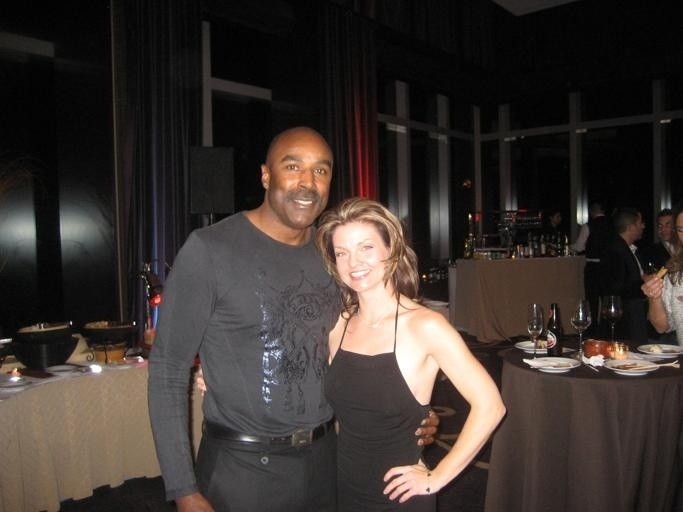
[358,305,396,329]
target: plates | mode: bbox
[530,357,580,373]
[0,359,82,393]
[604,359,658,375]
[513,339,550,355]
[636,343,683,358]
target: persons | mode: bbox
[148,127,439,512]
[542,198,683,346]
[197,198,507,512]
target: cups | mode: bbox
[583,340,607,357]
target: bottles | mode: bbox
[460,230,570,260]
[546,304,562,355]
[419,269,449,299]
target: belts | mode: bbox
[202,417,337,449]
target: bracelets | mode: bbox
[426,470,432,496]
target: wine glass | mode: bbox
[601,294,625,352]
[527,302,544,355]
[571,300,591,358]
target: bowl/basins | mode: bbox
[78,320,136,344]
[12,319,73,341]
